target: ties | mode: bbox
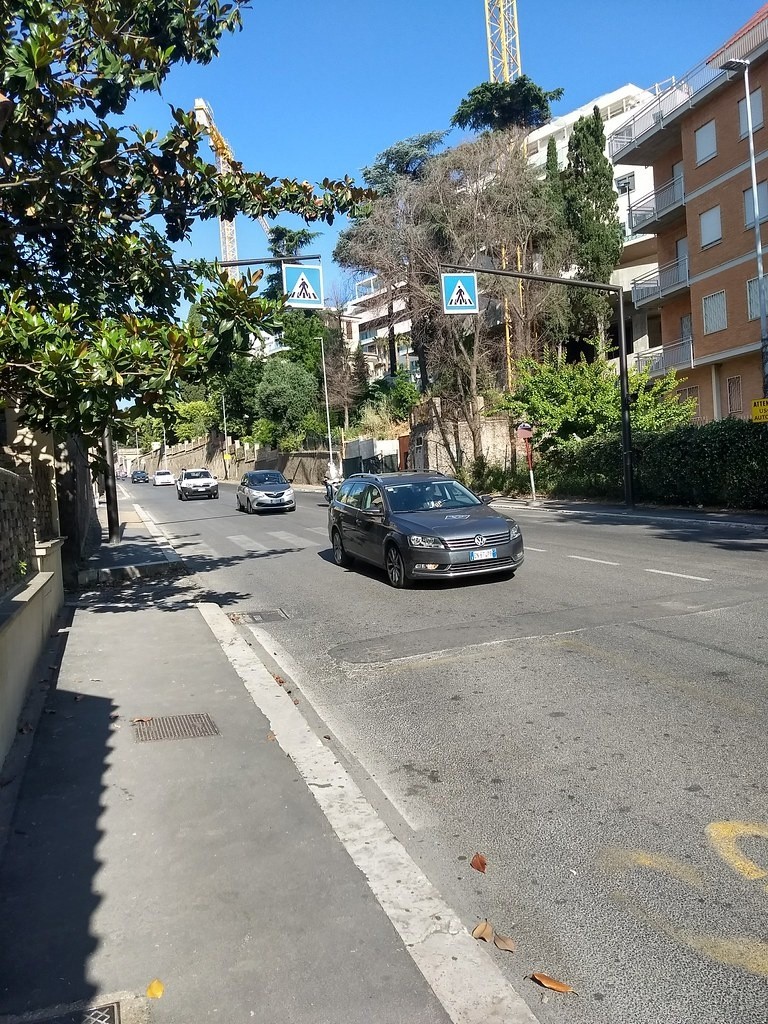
[428,500,432,508]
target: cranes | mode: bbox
[195,98,276,284]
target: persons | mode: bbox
[200,473,205,478]
[323,461,344,501]
[415,486,443,510]
[341,486,358,507]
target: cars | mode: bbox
[152,470,175,486]
[236,470,296,514]
[131,470,149,483]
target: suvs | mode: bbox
[177,468,219,500]
[328,469,525,589]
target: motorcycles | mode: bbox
[322,475,344,504]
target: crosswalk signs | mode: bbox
[441,273,480,315]
[281,263,324,310]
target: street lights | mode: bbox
[719,59,768,398]
[218,392,230,480]
[313,336,333,462]
